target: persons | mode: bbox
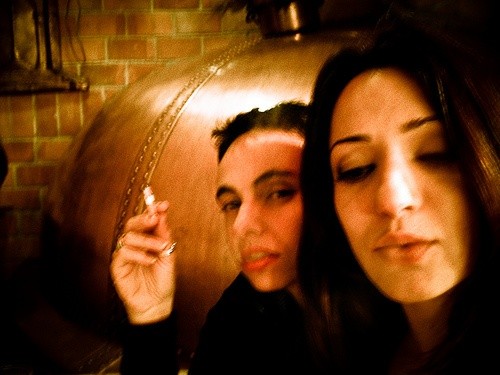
[99,96,313,374]
[302,30,500,374]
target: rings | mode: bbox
[159,242,179,257]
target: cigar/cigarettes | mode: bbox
[138,185,159,218]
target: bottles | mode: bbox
[12,6,38,72]
